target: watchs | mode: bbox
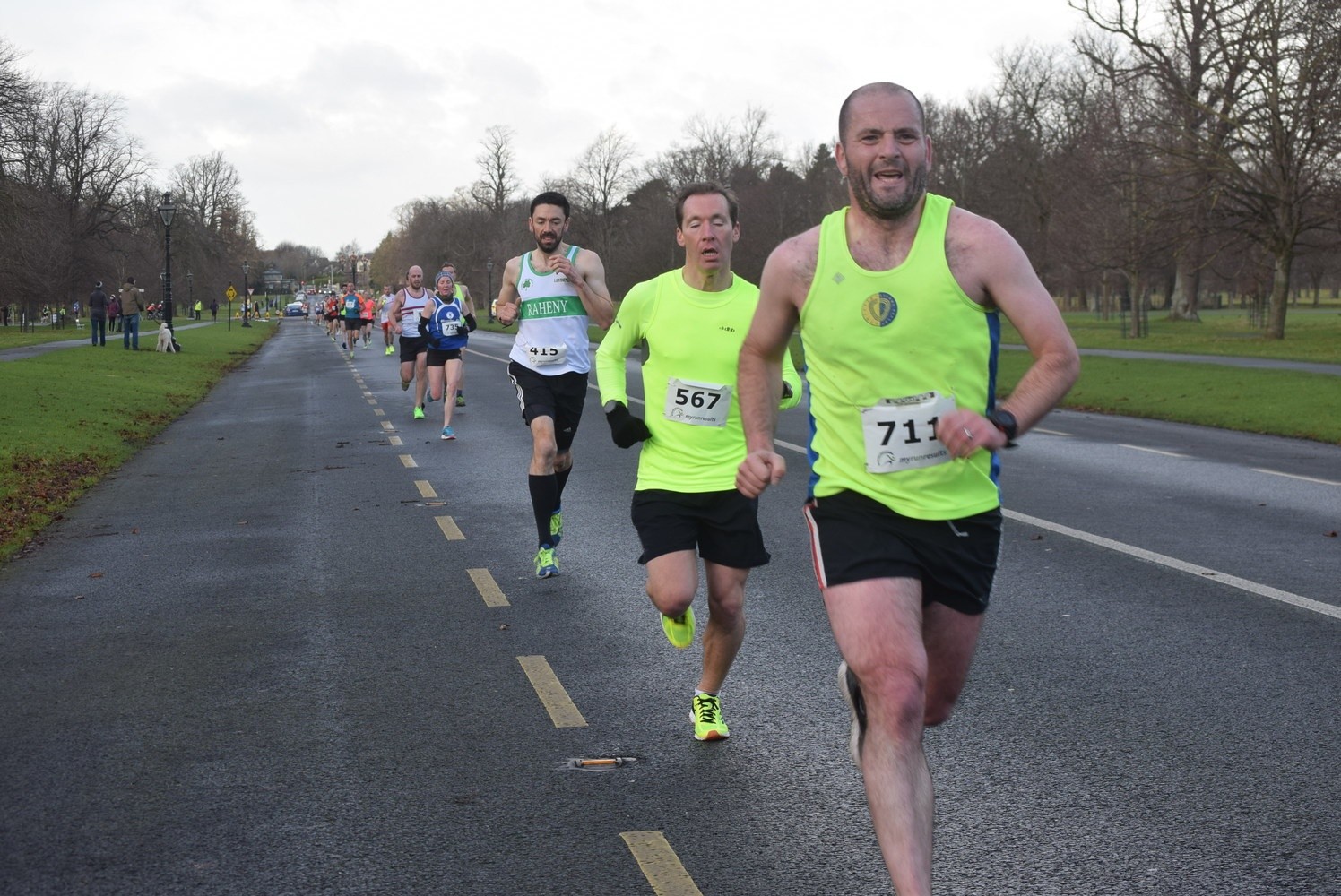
[987,407,1020,441]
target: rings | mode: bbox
[963,427,973,440]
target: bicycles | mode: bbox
[147,308,164,320]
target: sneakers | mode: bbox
[659,605,696,648]
[426,387,434,402]
[837,660,866,771]
[551,503,563,547]
[386,344,395,355]
[402,380,409,390]
[533,543,560,579]
[689,693,730,740]
[456,396,466,406]
[441,426,456,439]
[413,406,425,419]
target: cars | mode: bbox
[292,285,336,316]
[283,303,304,317]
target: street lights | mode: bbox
[485,257,495,324]
[157,190,181,352]
[240,259,252,327]
[186,270,195,319]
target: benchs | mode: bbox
[76,319,85,328]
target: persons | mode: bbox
[120,277,145,351]
[146,300,164,318]
[194,299,202,321]
[210,299,219,322]
[495,191,615,580]
[300,281,397,360]
[88,281,110,347]
[41,299,80,320]
[734,80,1083,896]
[108,292,123,333]
[388,262,477,440]
[594,182,804,743]
[241,299,261,319]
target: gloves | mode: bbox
[457,326,469,336]
[430,337,440,349]
[605,400,652,449]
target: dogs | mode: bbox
[156,322,176,353]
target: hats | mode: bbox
[110,294,116,298]
[96,281,103,288]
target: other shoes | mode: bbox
[363,344,367,349]
[342,343,347,349]
[331,336,337,342]
[349,351,354,358]
[368,339,372,345]
